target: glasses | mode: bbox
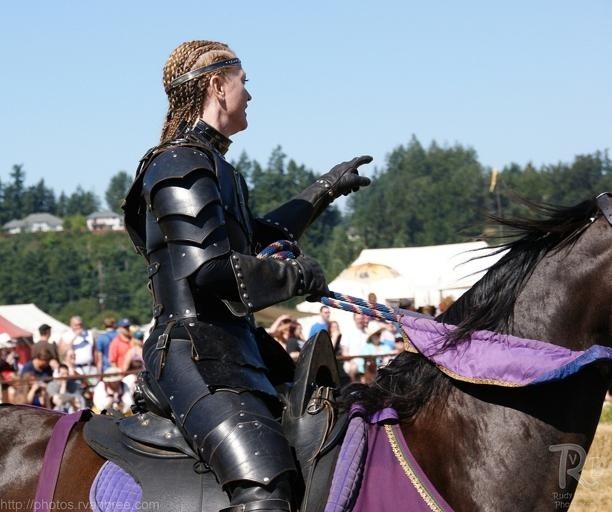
[395,338,403,343]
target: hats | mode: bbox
[117,318,144,338]
[365,319,386,343]
[102,367,121,383]
[38,324,51,330]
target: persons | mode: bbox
[0,303,445,412]
[119,40,374,511]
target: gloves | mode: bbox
[253,155,373,245]
[215,250,330,319]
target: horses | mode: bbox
[1,189,611,511]
